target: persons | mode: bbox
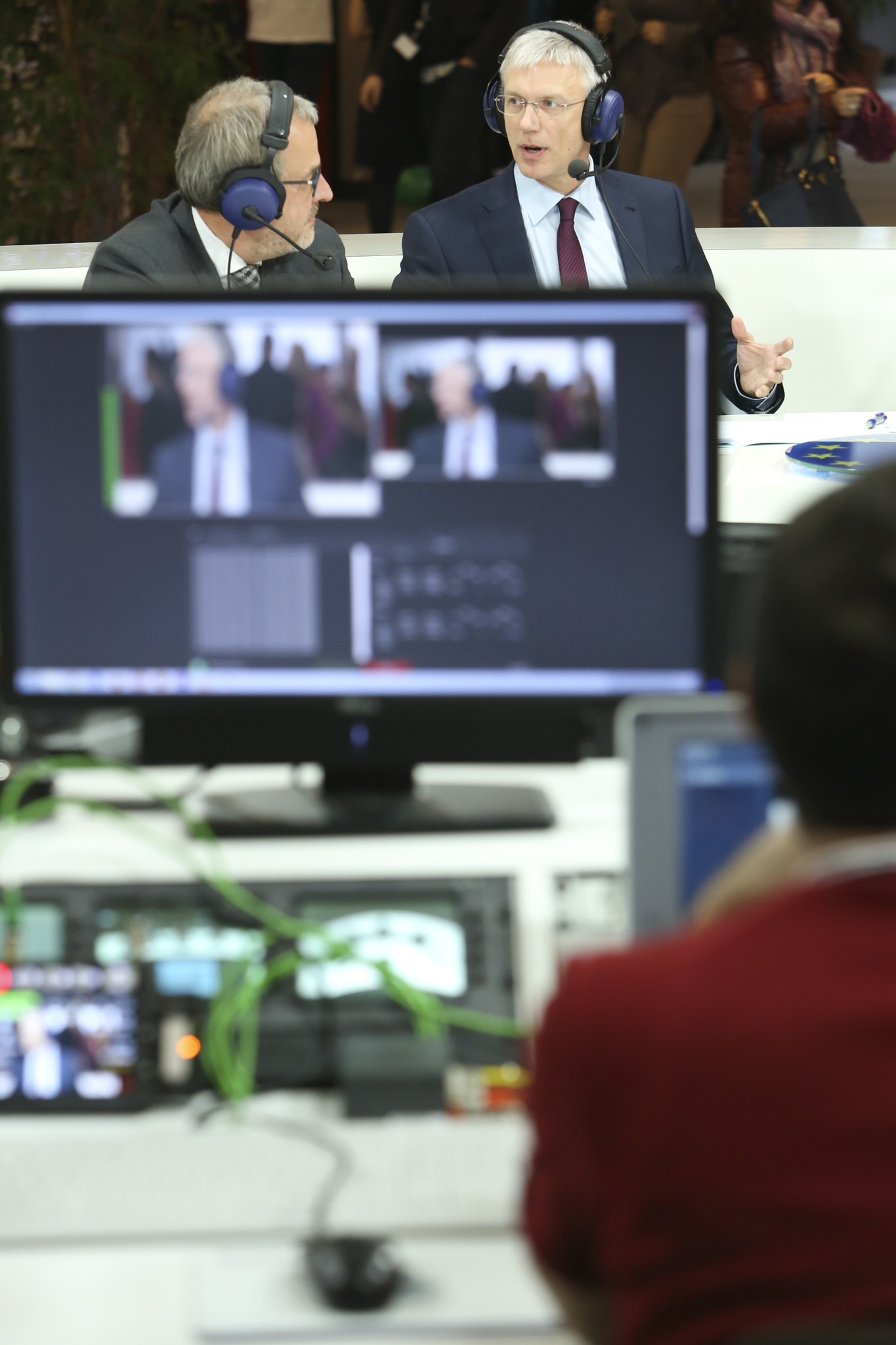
[504,466,895,1345]
[79,74,361,294]
[143,329,605,526]
[243,0,336,99]
[698,0,896,227]
[591,0,740,220]
[391,19,795,414]
[363,2,480,233]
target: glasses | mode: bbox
[280,167,322,197]
[494,95,586,120]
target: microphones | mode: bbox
[568,113,625,181]
[243,207,334,272]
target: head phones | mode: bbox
[217,78,296,231]
[484,22,625,156]
[215,328,243,398]
[470,360,487,403]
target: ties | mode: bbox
[458,426,474,484]
[555,196,591,290]
[206,444,223,520]
[230,265,262,292]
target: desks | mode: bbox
[712,411,895,577]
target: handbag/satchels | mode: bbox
[739,77,865,227]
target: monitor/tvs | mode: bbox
[1,283,717,765]
[619,695,792,933]
[0,877,515,1118]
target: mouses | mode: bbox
[310,1237,406,1311]
